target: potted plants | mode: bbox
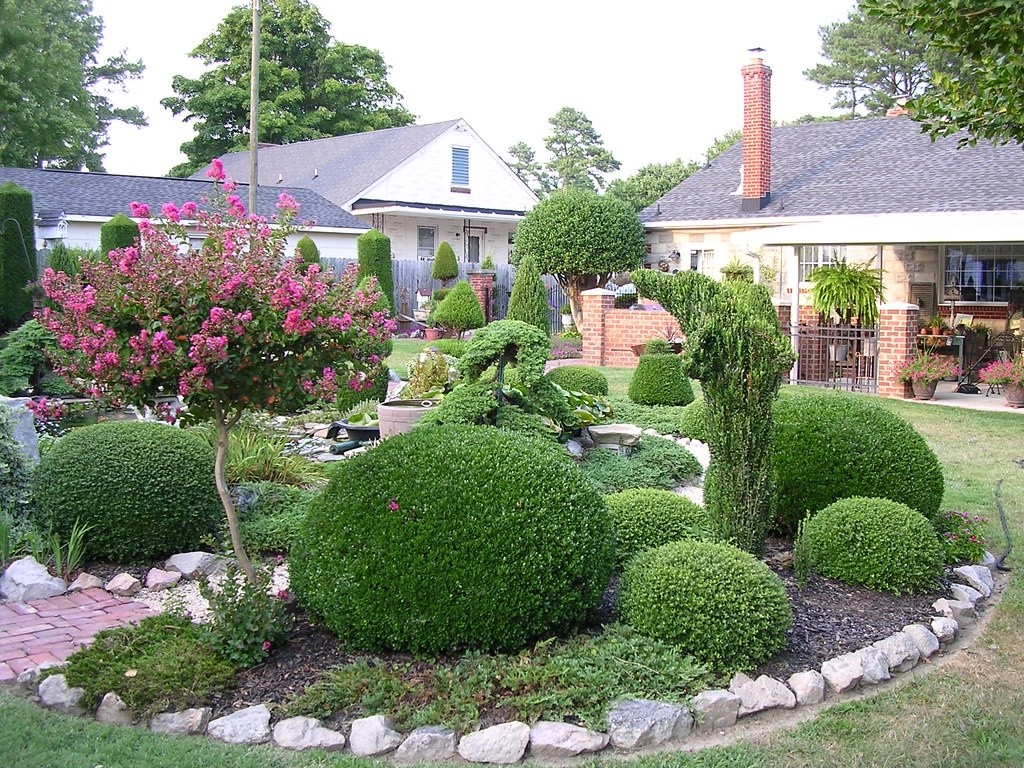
[929,311,985,334]
[807,250,888,361]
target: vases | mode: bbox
[911,376,938,399]
[920,328,928,334]
[1003,383,1024,408]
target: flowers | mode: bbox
[978,351,1024,389]
[884,350,965,381]
